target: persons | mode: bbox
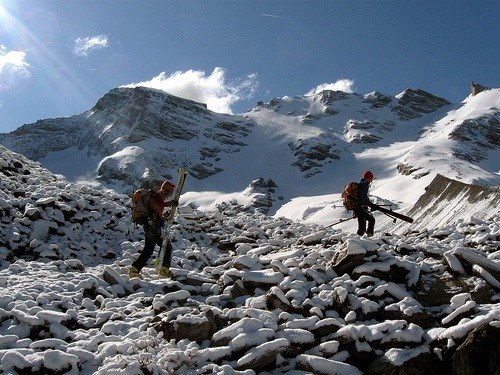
[128,178,179,279]
[350,170,377,237]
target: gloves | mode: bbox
[171,200,179,207]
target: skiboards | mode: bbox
[154,166,187,271]
[367,203,413,223]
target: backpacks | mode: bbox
[342,182,359,210]
[131,189,151,224]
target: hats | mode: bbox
[363,171,373,181]
[161,180,175,191]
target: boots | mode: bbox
[127,266,143,279]
[158,267,173,279]
